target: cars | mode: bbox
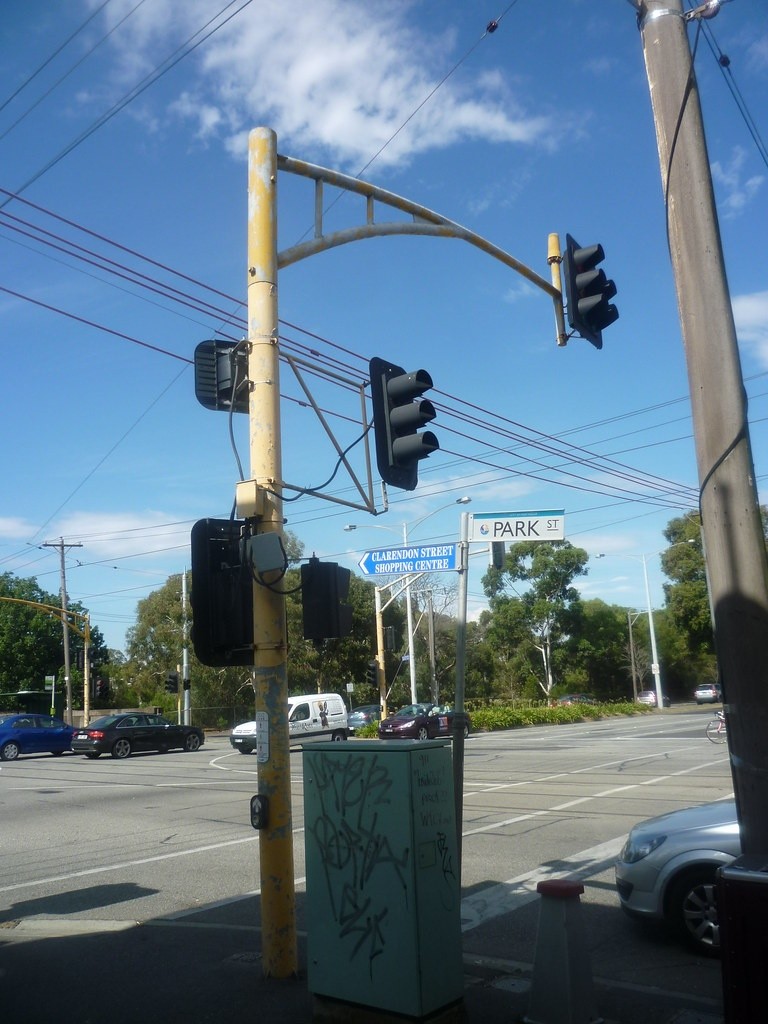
[379,704,473,740]
[71,712,205,758]
[554,694,598,706]
[348,704,394,730]
[636,690,671,708]
[0,715,79,761]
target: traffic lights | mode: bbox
[184,679,191,690]
[301,560,354,645]
[93,675,110,699]
[164,675,178,694]
[564,236,620,351]
[88,648,100,659]
[369,357,439,494]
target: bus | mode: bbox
[0,692,63,720]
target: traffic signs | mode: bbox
[470,508,565,543]
[360,542,462,575]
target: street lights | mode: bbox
[596,540,697,709]
[343,496,472,714]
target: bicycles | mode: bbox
[706,708,727,744]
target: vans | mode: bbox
[228,693,349,754]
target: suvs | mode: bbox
[694,683,722,704]
